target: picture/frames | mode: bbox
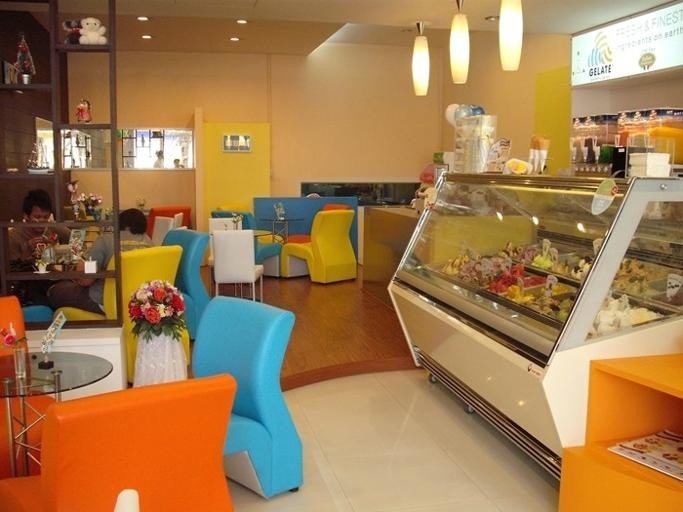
[220,133,252,154]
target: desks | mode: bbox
[0,349,111,476]
[359,202,534,313]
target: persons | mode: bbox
[5,188,71,306]
[153,149,165,168]
[174,159,184,168]
[48,209,152,316]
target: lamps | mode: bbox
[496,0,525,72]
[408,20,431,96]
[448,0,472,86]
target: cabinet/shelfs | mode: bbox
[60,129,89,170]
[386,167,683,483]
[556,351,683,512]
[1,0,127,330]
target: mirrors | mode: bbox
[34,116,195,176]
[298,181,423,207]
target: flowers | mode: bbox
[0,322,28,352]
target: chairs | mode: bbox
[189,296,304,498]
[20,206,209,384]
[0,296,62,478]
[0,371,240,511]
[209,197,356,302]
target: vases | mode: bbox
[11,347,26,379]
